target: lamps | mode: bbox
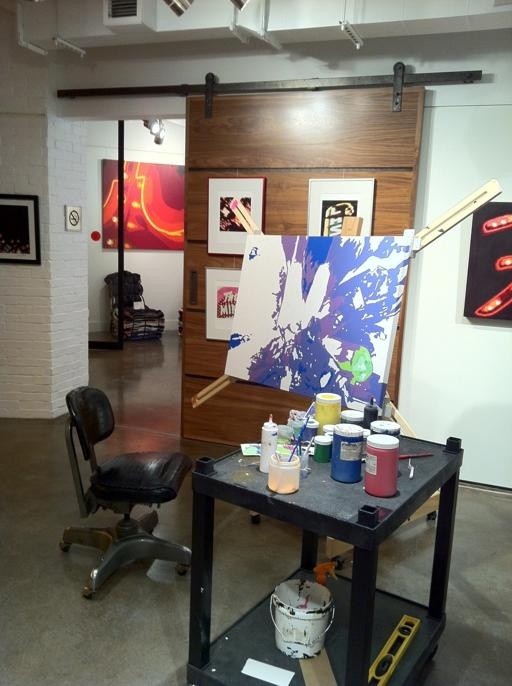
[141,119,166,144]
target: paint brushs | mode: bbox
[288,415,310,462]
[304,402,315,418]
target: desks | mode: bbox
[187,434,463,685]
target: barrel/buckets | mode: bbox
[269,578,337,660]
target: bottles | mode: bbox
[261,387,402,499]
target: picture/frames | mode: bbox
[206,176,266,256]
[0,194,41,264]
[205,266,241,342]
[306,178,375,236]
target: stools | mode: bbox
[178,307,184,337]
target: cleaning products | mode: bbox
[312,560,338,585]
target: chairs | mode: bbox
[60,386,192,600]
[104,271,165,341]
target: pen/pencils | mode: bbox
[399,450,435,460]
[293,434,315,462]
